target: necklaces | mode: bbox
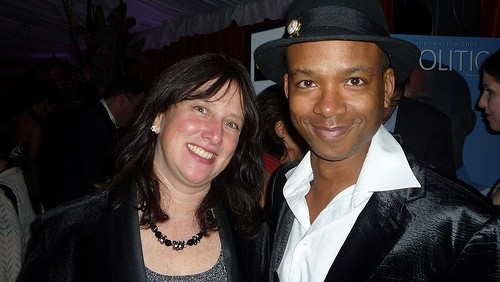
[134,187,214,249]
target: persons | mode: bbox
[1,37,150,282]
[253,0,500,282]
[250,84,310,212]
[394,58,475,182]
[476,50,500,212]
[376,79,457,185]
[14,52,272,282]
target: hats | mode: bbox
[254,0,422,86]
